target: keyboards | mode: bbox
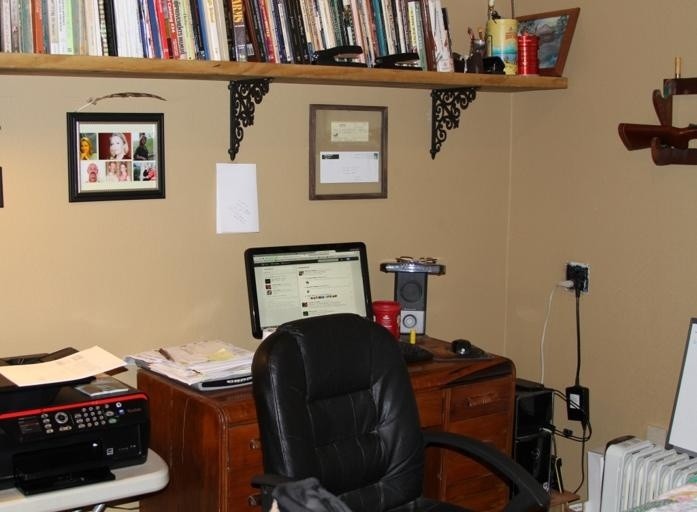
[398,340,435,363]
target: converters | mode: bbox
[565,385,590,421]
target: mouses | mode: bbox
[452,339,473,355]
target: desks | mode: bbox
[134,330,518,504]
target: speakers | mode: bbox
[395,272,427,335]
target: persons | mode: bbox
[107,161,118,181]
[86,164,99,181]
[119,163,130,181]
[108,133,131,159]
[80,137,96,161]
[143,162,155,181]
[134,136,149,160]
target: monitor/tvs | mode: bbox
[244,240,375,339]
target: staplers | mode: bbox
[374,53,423,70]
[311,46,367,66]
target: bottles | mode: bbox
[410,329,416,344]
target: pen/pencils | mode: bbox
[159,348,176,362]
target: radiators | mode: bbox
[601,430,696,510]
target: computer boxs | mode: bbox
[511,390,554,497]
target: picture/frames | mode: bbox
[66,110,166,201]
[308,102,391,201]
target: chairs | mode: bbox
[257,312,550,512]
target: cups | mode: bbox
[372,301,402,341]
[471,18,539,76]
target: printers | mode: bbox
[0,346,152,496]
